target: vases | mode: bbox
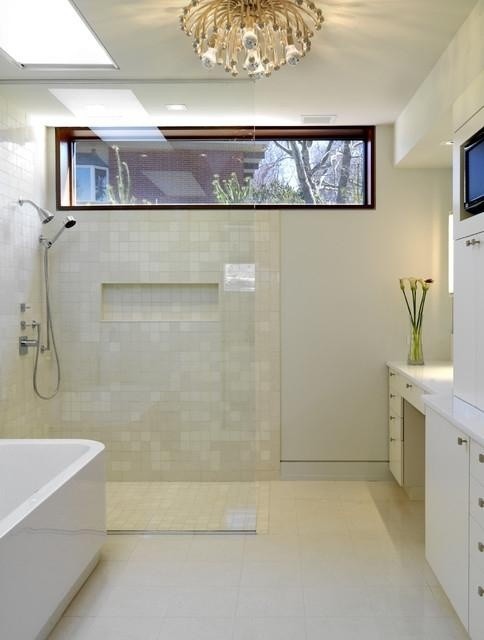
[405,330,424,366]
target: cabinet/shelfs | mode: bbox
[401,376,428,416]
[424,404,470,625]
[468,438,484,624]
[454,232,483,416]
[387,368,424,489]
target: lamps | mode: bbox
[177,0,322,84]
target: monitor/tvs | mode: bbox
[458,126,484,218]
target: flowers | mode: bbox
[395,272,435,366]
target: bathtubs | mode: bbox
[1,438,107,640]
